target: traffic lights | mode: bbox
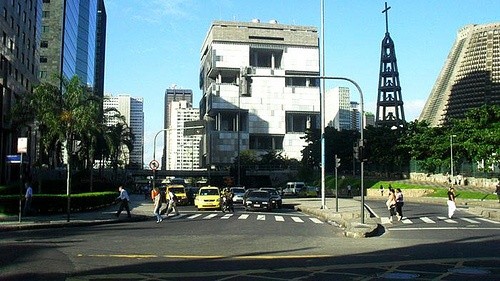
[336,158,341,168]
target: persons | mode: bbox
[447,188,456,219]
[380,185,384,196]
[221,186,234,213]
[164,190,178,215]
[347,184,352,198]
[386,189,402,222]
[150,188,163,224]
[114,186,131,218]
[21,181,41,215]
[396,188,403,222]
[315,186,320,198]
[144,183,149,199]
[139,184,143,195]
[495,179,500,203]
[388,183,393,191]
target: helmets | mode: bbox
[226,187,230,189]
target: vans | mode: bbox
[282,182,306,195]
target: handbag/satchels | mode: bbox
[173,193,176,200]
[388,199,396,210]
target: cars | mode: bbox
[146,184,198,206]
[222,186,283,212]
[194,186,221,211]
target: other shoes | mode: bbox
[396,215,401,221]
[157,218,163,223]
[128,215,130,217]
[115,213,119,217]
[389,216,393,222]
